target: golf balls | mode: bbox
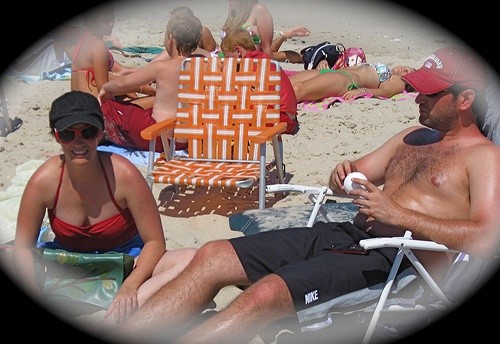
[343,171,369,197]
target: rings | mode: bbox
[127,298,131,302]
[113,299,118,303]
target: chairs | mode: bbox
[229,80,500,344]
[140,57,288,209]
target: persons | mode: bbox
[16,0,421,152]
[100,45,500,344]
[0,90,202,337]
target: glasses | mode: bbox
[53,125,103,145]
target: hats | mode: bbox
[49,91,105,134]
[400,45,495,95]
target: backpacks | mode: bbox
[301,40,345,70]
